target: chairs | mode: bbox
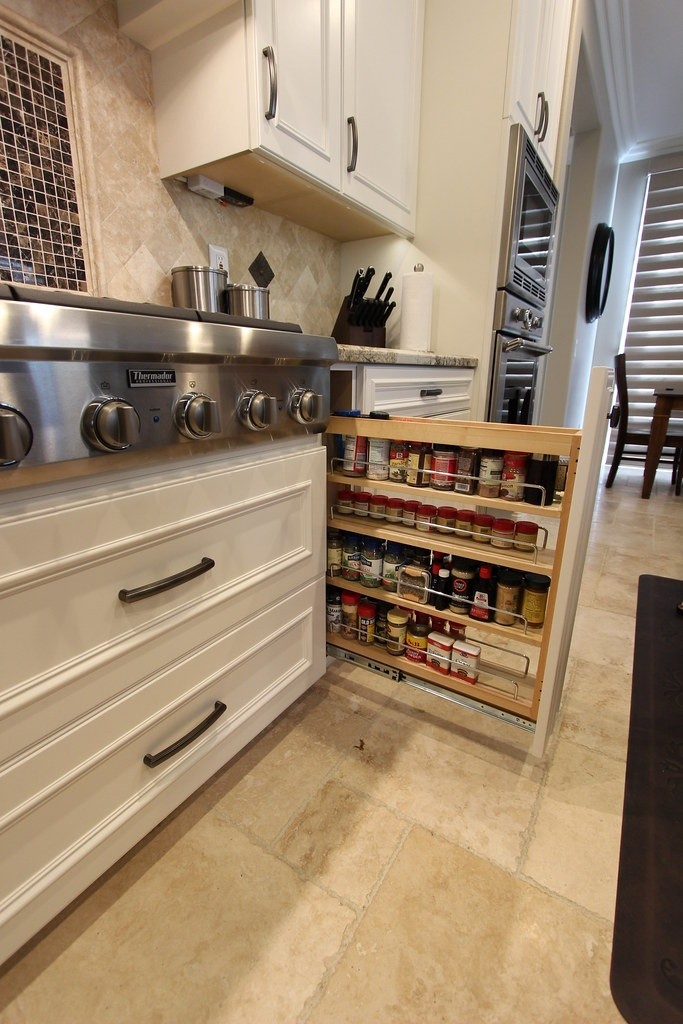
[606,353,683,495]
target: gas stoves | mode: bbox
[0,280,339,471]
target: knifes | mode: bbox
[348,266,396,328]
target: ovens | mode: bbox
[488,122,560,425]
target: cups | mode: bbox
[226,284,270,320]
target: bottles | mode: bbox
[436,568,451,610]
[468,565,496,622]
[341,590,409,655]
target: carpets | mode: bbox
[608,575,683,1024]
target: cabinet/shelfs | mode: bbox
[325,409,583,723]
[120,0,585,242]
[0,432,330,979]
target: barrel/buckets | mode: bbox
[171,266,228,314]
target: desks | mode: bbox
[641,380,683,497]
